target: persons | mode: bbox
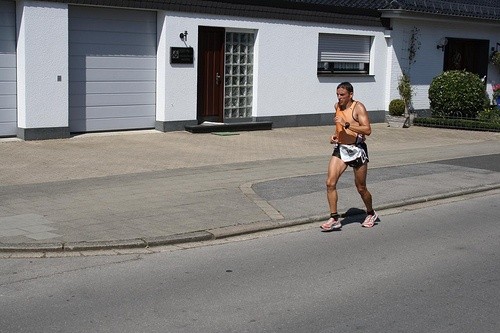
[315,81,378,229]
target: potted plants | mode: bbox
[386,99,409,128]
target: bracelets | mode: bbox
[344,121,350,130]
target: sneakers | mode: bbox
[319,217,342,231]
[361,210,379,228]
